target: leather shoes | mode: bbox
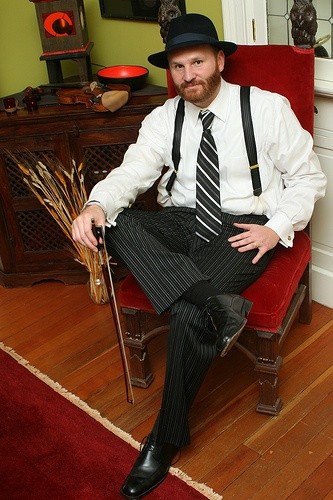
[120,433,181,500]
[198,292,253,357]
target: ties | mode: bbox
[196,109,223,242]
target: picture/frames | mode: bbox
[96,0,187,22]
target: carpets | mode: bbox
[0,339,224,500]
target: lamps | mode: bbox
[30,1,94,87]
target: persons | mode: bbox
[72,13,326,499]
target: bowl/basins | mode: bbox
[97,65,149,92]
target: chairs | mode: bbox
[114,43,315,416]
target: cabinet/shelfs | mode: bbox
[0,89,169,286]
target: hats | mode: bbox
[148,14,238,69]
